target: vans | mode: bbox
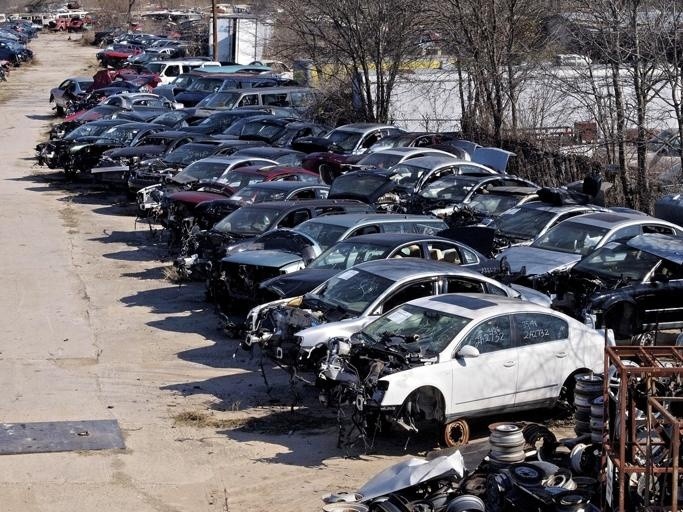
[154,73,258,96]
[556,53,593,65]
[153,64,220,81]
[194,87,316,114]
[168,64,273,91]
[177,79,297,105]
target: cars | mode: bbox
[233,146,306,161]
[135,164,323,213]
[327,126,407,153]
[91,12,205,57]
[127,49,164,75]
[204,214,449,309]
[356,138,476,176]
[278,117,327,149]
[315,293,612,448]
[130,141,266,185]
[75,123,171,172]
[563,233,683,336]
[203,200,371,255]
[170,157,277,187]
[37,118,162,169]
[175,182,331,259]
[92,130,223,190]
[49,76,93,111]
[93,141,226,198]
[2,10,52,43]
[76,91,183,115]
[245,258,552,374]
[196,107,269,133]
[435,185,538,222]
[0,42,33,61]
[388,146,516,203]
[488,200,605,255]
[420,172,537,196]
[494,196,683,278]
[96,42,137,66]
[250,59,294,80]
[218,114,297,143]
[255,232,509,302]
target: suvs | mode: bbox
[44,8,88,31]
